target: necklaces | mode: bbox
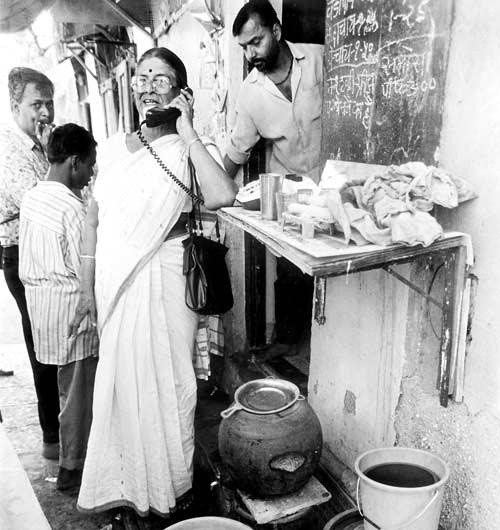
[270,53,296,86]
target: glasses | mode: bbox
[128,73,179,95]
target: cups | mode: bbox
[260,172,283,222]
[274,192,294,224]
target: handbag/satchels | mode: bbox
[182,146,235,317]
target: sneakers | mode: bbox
[57,466,82,491]
[41,443,62,460]
[250,342,299,360]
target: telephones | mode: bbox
[144,88,193,128]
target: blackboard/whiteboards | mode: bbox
[325,0,452,169]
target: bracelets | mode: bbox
[185,138,199,157]
[78,254,96,261]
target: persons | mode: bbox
[16,124,98,495]
[219,2,325,184]
[63,48,239,521]
[1,67,62,459]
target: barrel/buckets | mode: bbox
[166,516,251,530]
[355,447,451,530]
